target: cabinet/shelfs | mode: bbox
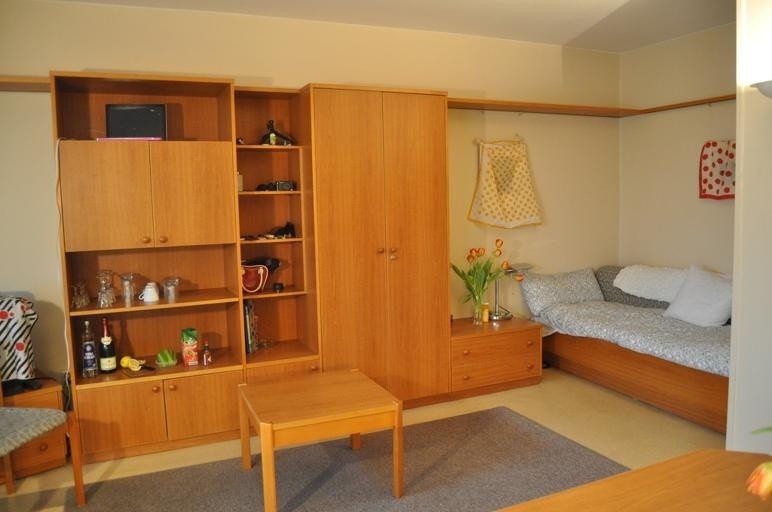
[47,69,246,467]
[0,379,65,486]
[233,84,312,438]
[302,83,448,412]
[451,316,542,402]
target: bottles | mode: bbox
[203,341,212,367]
[269,120,277,145]
[99,318,117,373]
[81,321,100,378]
[481,302,489,322]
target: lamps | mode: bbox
[489,265,531,322]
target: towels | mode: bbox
[697,139,736,200]
[467,138,543,229]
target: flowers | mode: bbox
[745,426,772,501]
[450,237,524,299]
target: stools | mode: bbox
[0,405,86,505]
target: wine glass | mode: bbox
[70,281,90,309]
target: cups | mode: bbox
[139,286,159,302]
[119,271,135,306]
[147,282,160,297]
[96,269,117,308]
[164,278,180,299]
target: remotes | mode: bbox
[23,378,42,390]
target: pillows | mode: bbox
[662,265,734,328]
[519,267,606,316]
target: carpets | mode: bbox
[0,406,632,512]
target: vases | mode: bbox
[472,298,483,326]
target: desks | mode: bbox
[238,368,403,512]
[493,446,772,512]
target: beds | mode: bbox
[531,263,730,435]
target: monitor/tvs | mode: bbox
[106,102,168,140]
